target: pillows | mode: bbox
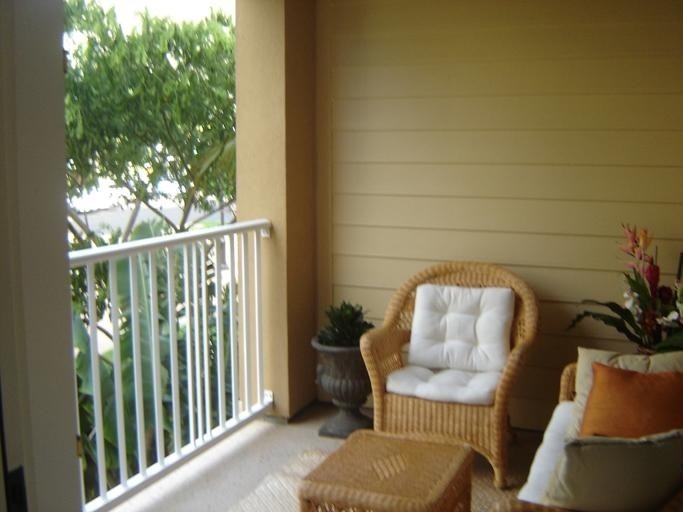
[514,343,682,506]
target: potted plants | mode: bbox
[312,299,373,436]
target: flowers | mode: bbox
[563,220,683,349]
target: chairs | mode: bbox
[359,252,540,489]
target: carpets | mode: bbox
[220,424,545,509]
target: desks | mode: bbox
[298,428,471,509]
